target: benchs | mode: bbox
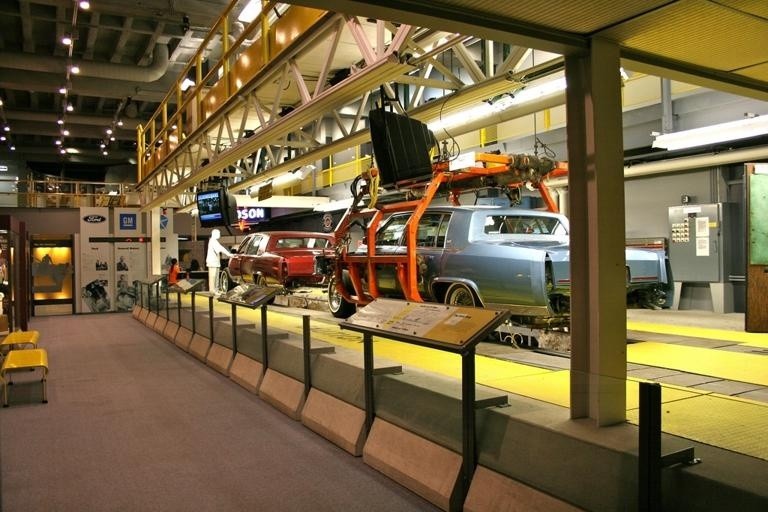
[0,331,39,372]
[0,349,49,408]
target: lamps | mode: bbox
[100,100,125,155]
[0,100,16,151]
[55,0,90,156]
[146,119,178,158]
[179,60,210,92]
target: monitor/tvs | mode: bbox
[368,108,436,187]
[196,188,238,228]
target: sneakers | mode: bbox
[212,290,226,297]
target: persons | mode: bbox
[0,252,8,297]
[189,259,204,271]
[356,236,368,253]
[159,207,169,230]
[205,227,236,293]
[83,254,135,311]
[200,197,219,214]
[168,258,180,287]
[228,21,254,72]
